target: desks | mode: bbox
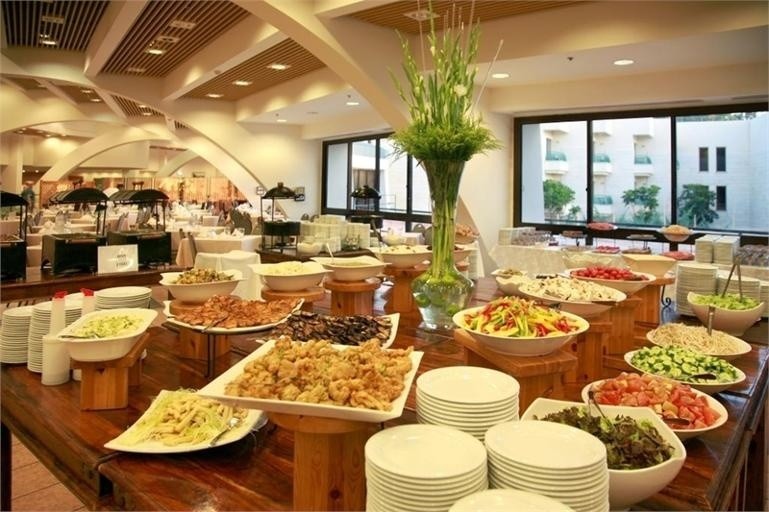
[1,268,769,511]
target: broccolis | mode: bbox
[224,332,419,409]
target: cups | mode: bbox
[224,228,231,239]
[237,228,245,239]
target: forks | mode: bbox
[210,416,241,444]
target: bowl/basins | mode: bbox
[562,255,620,269]
[309,255,392,282]
[581,378,729,442]
[686,292,766,337]
[521,397,687,512]
[247,262,334,292]
[620,254,677,277]
[496,275,532,297]
[490,269,528,280]
[424,246,479,265]
[56,307,159,363]
[645,330,752,362]
[158,269,248,304]
[376,245,432,269]
[562,267,657,298]
[518,285,627,317]
[623,350,746,395]
[452,306,589,357]
[655,229,697,242]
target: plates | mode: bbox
[444,485,578,512]
[415,365,521,447]
[166,298,305,335]
[585,225,619,231]
[94,286,152,361]
[717,275,761,303]
[195,339,425,424]
[674,263,720,317]
[0,306,31,364]
[760,280,769,318]
[26,301,82,373]
[364,423,488,512]
[300,214,371,250]
[103,389,264,455]
[484,420,610,512]
[625,235,657,241]
[694,233,740,266]
[255,312,400,349]
[498,227,536,246]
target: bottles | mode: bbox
[72,288,94,381]
[41,290,70,386]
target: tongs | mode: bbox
[706,305,715,337]
[721,256,743,301]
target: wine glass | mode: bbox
[519,230,552,248]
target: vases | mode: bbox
[412,158,475,332]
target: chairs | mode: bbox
[188,233,196,264]
[178,229,186,241]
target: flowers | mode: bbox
[382,1,504,288]
